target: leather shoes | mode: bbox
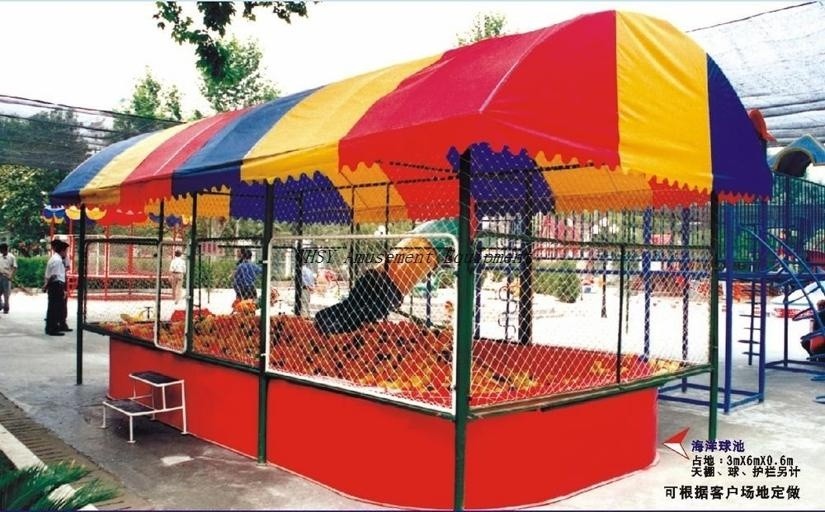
[60,327,73,331]
[49,331,64,336]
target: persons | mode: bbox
[50,239,72,331]
[39,241,69,336]
[800,300,825,361]
[0,242,18,314]
[301,258,317,319]
[230,247,263,301]
[167,249,186,304]
